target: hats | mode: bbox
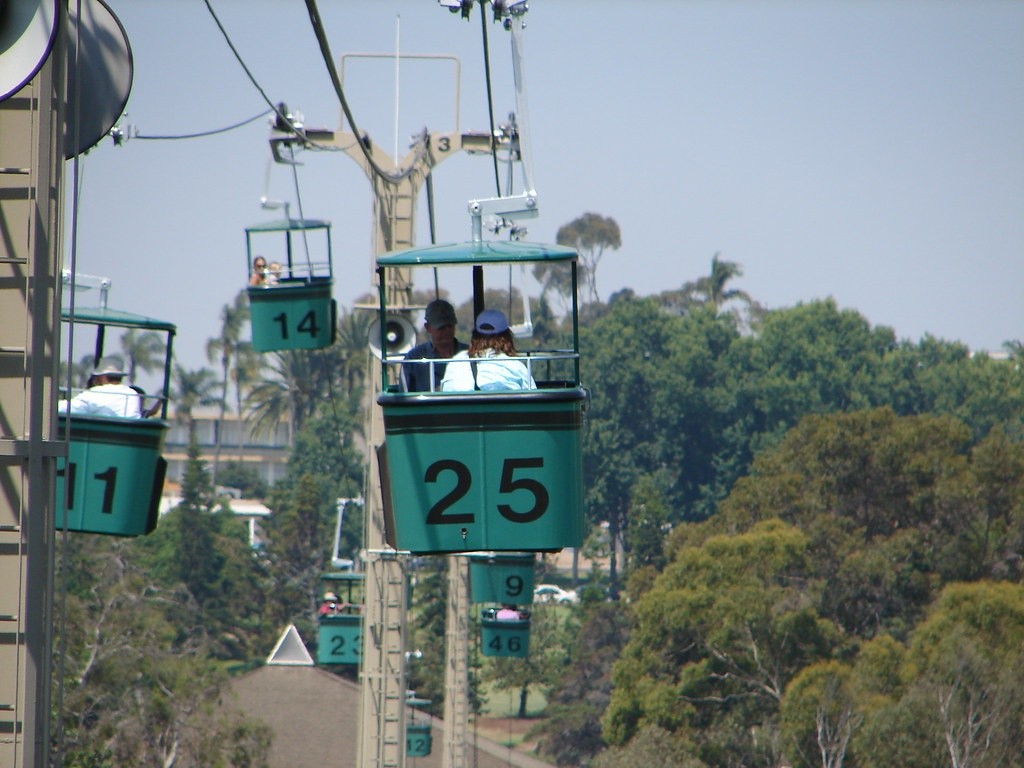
[425,300,458,329]
[324,592,337,602]
[263,263,285,273]
[90,357,129,376]
[476,309,509,334]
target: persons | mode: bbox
[398,298,472,391]
[58,354,161,419]
[320,588,525,620]
[440,309,539,392]
[248,257,282,286]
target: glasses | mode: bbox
[256,264,267,268]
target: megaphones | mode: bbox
[368,314,417,362]
